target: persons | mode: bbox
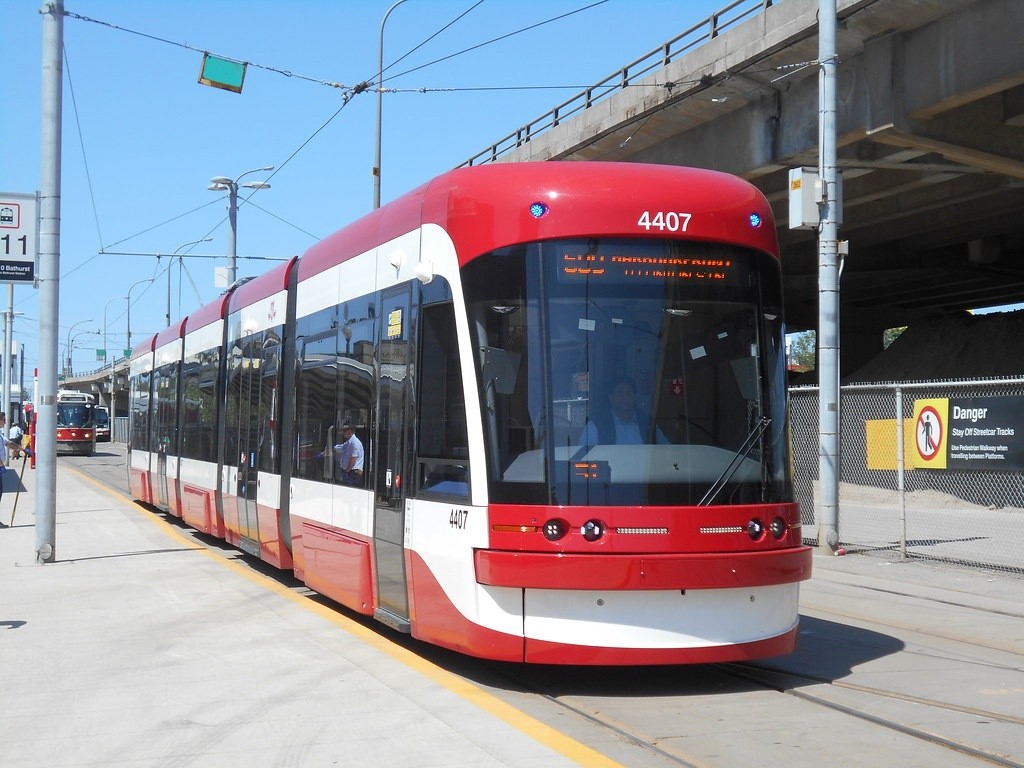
[0,412,31,529]
[339,422,365,488]
[577,374,671,444]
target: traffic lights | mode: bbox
[25,402,33,414]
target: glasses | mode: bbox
[0,418,5,422]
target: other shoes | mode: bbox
[0,522,9,528]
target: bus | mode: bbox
[126,161,813,666]
[57,388,99,456]
[96,405,111,442]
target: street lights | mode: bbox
[1,311,25,415]
[207,165,275,288]
[165,237,214,327]
[96,296,131,371]
[123,274,154,359]
[61,331,90,377]
[67,318,93,377]
